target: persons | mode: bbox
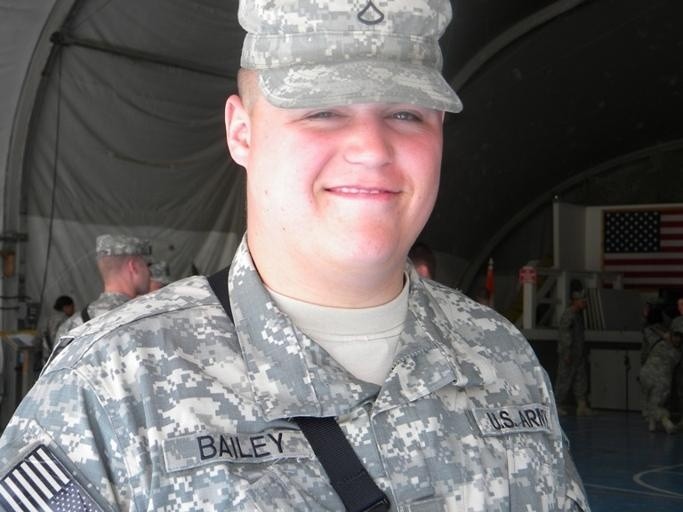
[670,299,682,341]
[473,285,492,308]
[636,303,682,435]
[50,233,154,356]
[39,294,76,369]
[141,259,170,294]
[0,0,590,512]
[551,287,598,418]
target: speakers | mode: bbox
[577,400,601,416]
[662,415,677,434]
[648,415,659,432]
[555,404,567,417]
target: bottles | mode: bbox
[150,261,171,284]
[94,235,152,257]
[572,288,589,301]
[237,1,465,115]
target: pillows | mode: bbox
[670,331,683,347]
